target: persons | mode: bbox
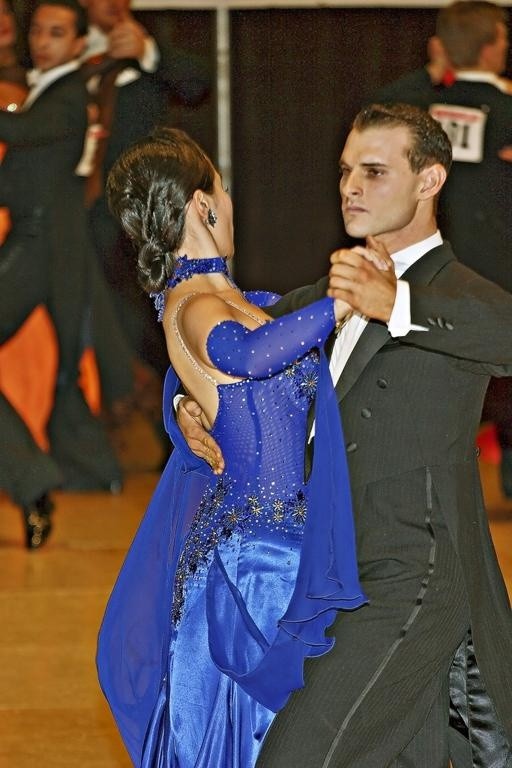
[170,101,509,768]
[94,122,394,766]
[2,1,218,551]
[365,2,512,500]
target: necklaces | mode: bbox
[166,251,229,288]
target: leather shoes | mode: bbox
[22,495,56,550]
